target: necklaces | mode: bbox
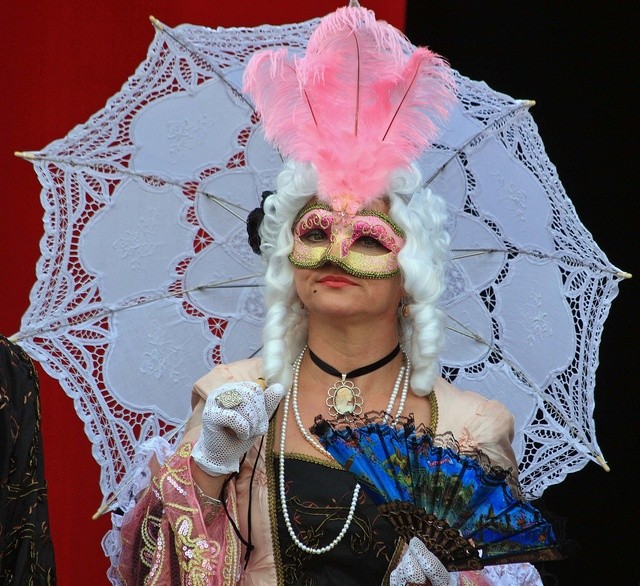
[309,342,402,424]
[279,344,411,555]
[292,343,406,462]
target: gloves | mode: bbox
[188,378,283,478]
[390,536,457,585]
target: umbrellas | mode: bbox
[3,0,634,586]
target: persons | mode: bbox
[116,157,550,586]
